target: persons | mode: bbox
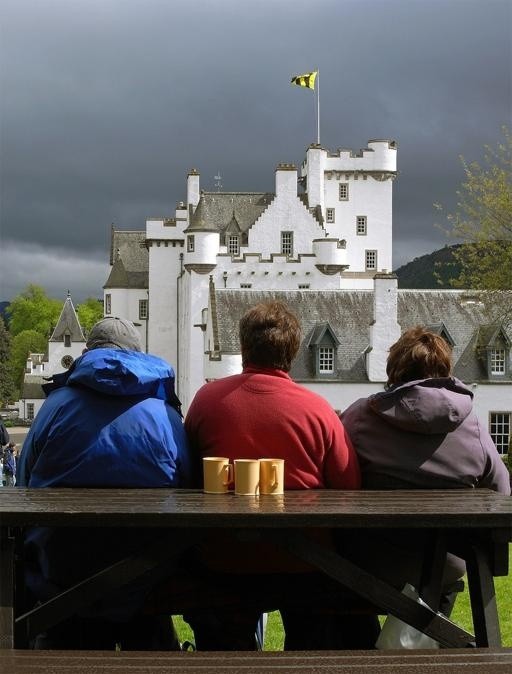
[338,325,509,650]
[184,299,362,651]
[0,414,20,486]
[15,316,196,651]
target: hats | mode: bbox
[86,316,142,353]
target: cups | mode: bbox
[233,459,260,495]
[202,456,234,494]
[260,459,284,494]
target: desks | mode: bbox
[0,484,512,646]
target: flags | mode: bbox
[290,72,318,90]
